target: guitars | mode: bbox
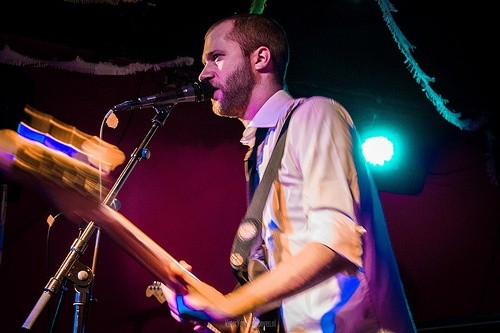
[1,107,283,332]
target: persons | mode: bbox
[162,12,418,333]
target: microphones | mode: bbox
[109,198,121,211]
[114,80,214,112]
